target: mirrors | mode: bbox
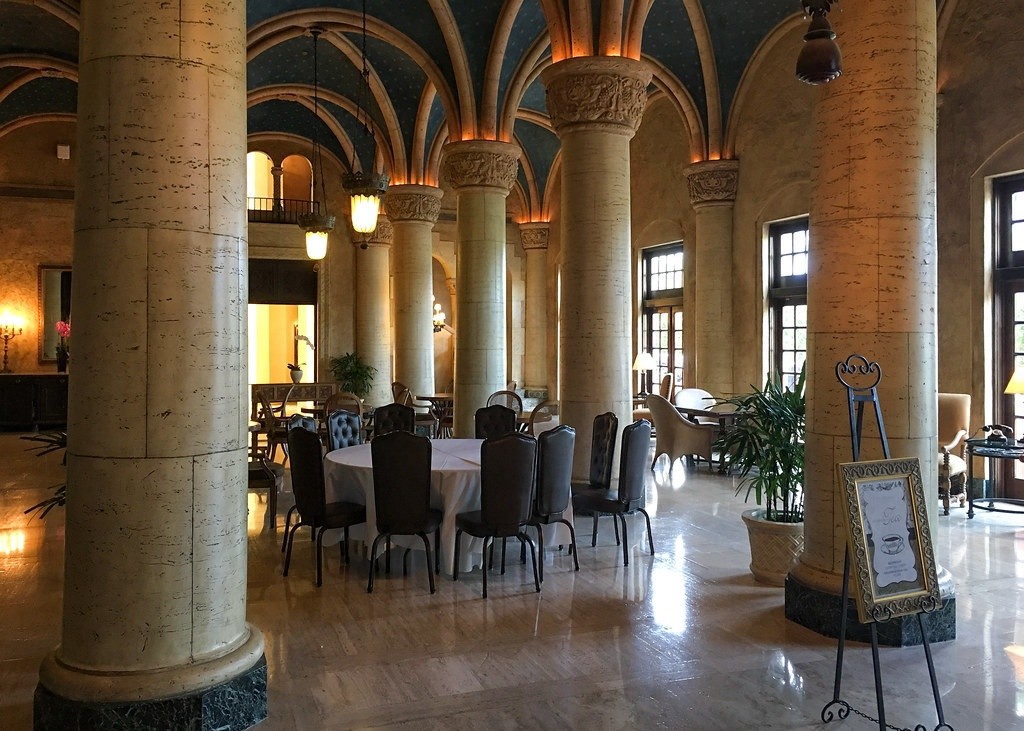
[37,265,72,365]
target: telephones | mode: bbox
[982,425,1013,442]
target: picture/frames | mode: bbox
[836,457,944,625]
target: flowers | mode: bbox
[53,318,70,352]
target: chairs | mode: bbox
[936,392,972,516]
[247,372,720,601]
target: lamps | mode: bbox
[339,0,390,249]
[0,321,23,373]
[297,25,336,261]
[1004,367,1024,444]
[632,353,657,398]
[794,0,844,86]
[432,294,446,333]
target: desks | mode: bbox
[301,402,372,452]
[515,411,552,435]
[318,438,576,567]
[251,382,336,461]
[415,392,453,440]
[965,437,1024,519]
[675,402,756,474]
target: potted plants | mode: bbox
[287,362,306,384]
[327,351,377,415]
[709,360,808,587]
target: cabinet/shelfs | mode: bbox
[0,373,69,433]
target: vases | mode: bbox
[56,347,69,373]
